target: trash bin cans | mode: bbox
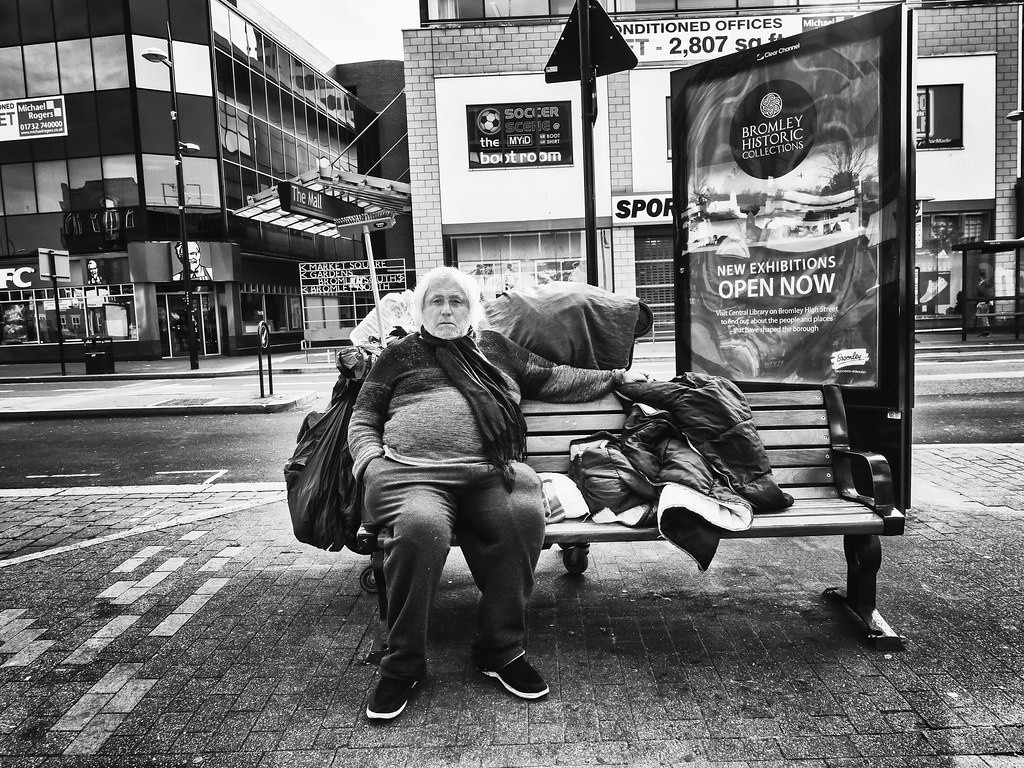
[83,337,116,374]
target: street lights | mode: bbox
[140,47,199,370]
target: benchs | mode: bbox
[357,383,906,661]
[974,310,1024,335]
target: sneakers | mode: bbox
[479,658,549,700]
[366,667,427,719]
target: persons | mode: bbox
[346,265,657,720]
[975,270,994,338]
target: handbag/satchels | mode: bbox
[285,382,361,551]
[570,431,647,515]
